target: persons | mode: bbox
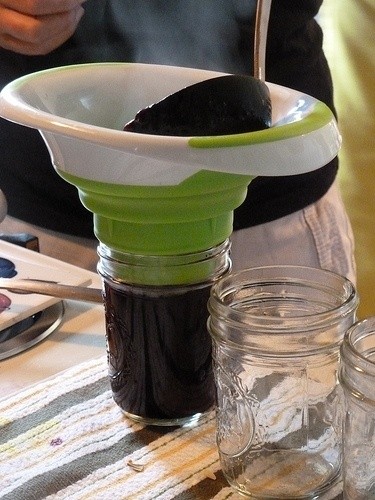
[0,0,358,323]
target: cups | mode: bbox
[337,314,375,500]
[206,263,359,500]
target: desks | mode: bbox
[0,240,344,500]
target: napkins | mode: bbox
[0,355,344,500]
